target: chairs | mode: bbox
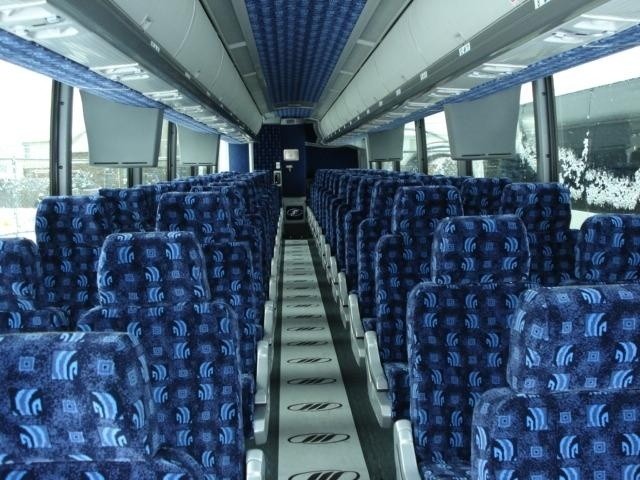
[1,324,210,480]
[77,226,266,480]
[466,280,640,480]
[1,170,285,440]
[387,208,558,480]
[304,166,640,430]
